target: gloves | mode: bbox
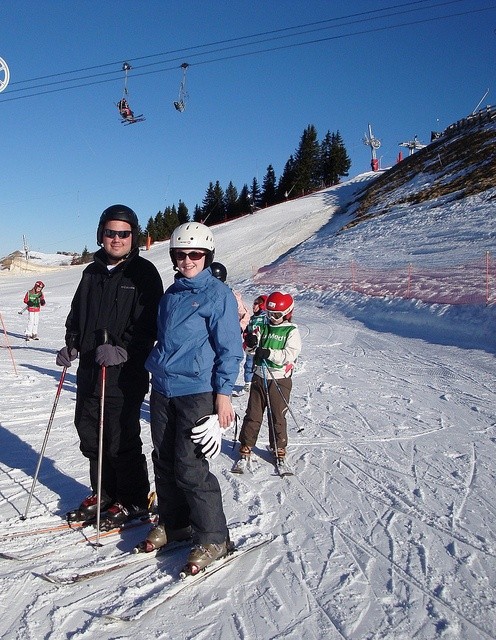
[95,344,128,367]
[256,347,271,359]
[200,411,235,459]
[190,403,234,443]
[56,346,78,367]
[27,300,33,306]
[40,299,45,305]
[245,332,258,348]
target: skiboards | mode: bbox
[228,444,293,475]
[31,522,273,620]
[0,491,159,560]
[231,389,246,396]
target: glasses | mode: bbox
[252,300,259,304]
[170,249,212,260]
[100,226,136,239]
[265,310,285,321]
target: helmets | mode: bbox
[266,292,294,319]
[168,222,215,251]
[256,293,269,312]
[210,262,227,282]
[34,281,44,289]
[97,204,139,244]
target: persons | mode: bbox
[206,258,251,391]
[141,222,238,572]
[58,206,165,525]
[116,97,137,124]
[243,295,270,390]
[21,281,47,341]
[232,293,301,475]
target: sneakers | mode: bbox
[104,501,148,520]
[144,523,192,551]
[186,539,229,571]
[80,492,110,514]
[239,444,251,456]
[273,447,285,457]
